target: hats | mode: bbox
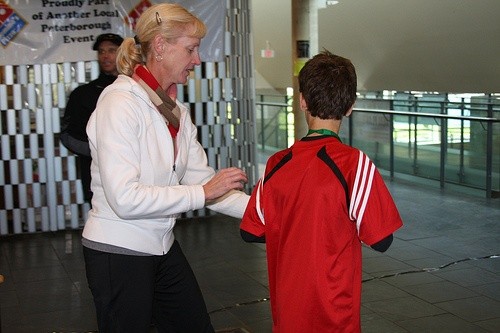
[93,32,124,50]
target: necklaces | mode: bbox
[306,129,342,142]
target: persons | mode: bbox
[59,33,124,210]
[239,47,404,333]
[81,3,252,333]
